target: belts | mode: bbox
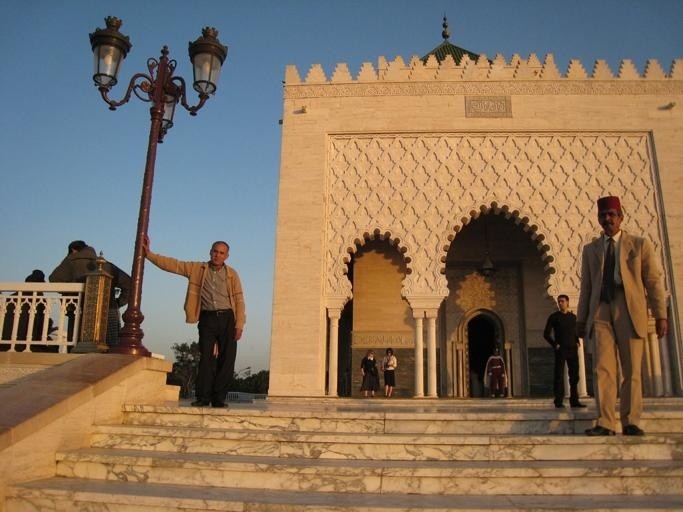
[201,311,225,318]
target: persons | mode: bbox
[482,346,507,398]
[543,295,586,407]
[380,348,397,397]
[0,270,58,352]
[360,350,380,397]
[48,240,131,352]
[576,196,669,437]
[141,236,246,407]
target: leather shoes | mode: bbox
[554,403,564,408]
[212,402,228,407]
[585,426,615,436]
[191,400,208,406]
[570,404,586,408]
[623,425,642,435]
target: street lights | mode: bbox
[87,16,229,356]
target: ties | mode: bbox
[599,237,614,304]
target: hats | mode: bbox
[597,196,620,211]
